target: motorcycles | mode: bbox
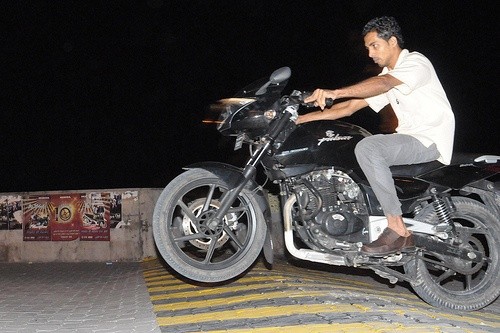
[152,67,499,308]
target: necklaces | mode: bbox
[390,86,402,106]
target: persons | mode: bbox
[294,16,456,254]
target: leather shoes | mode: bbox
[359,227,415,256]
